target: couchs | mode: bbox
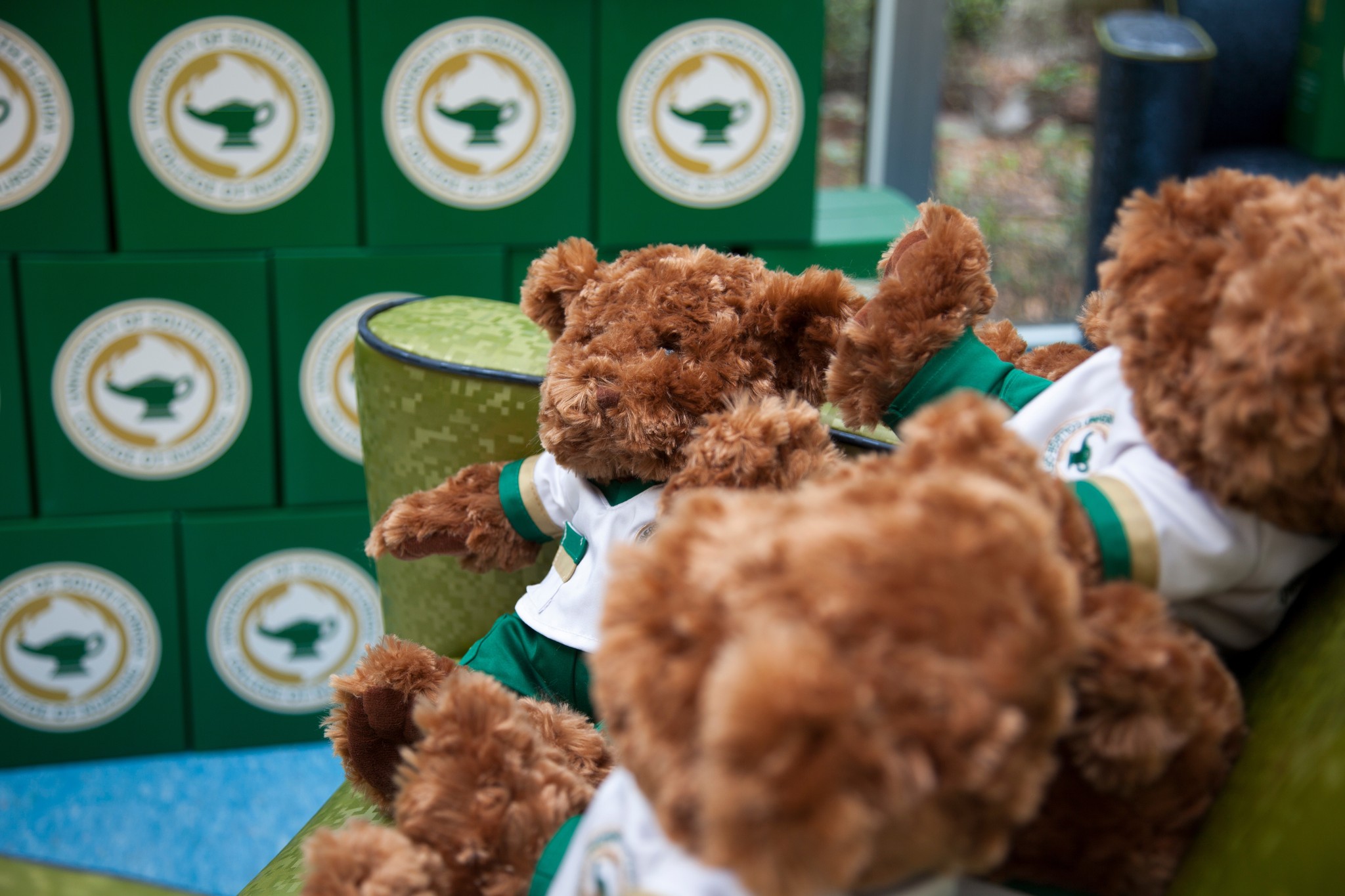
[0,284,1345,896]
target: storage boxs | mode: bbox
[0,0,924,769]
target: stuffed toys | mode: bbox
[293,163,1345,896]
